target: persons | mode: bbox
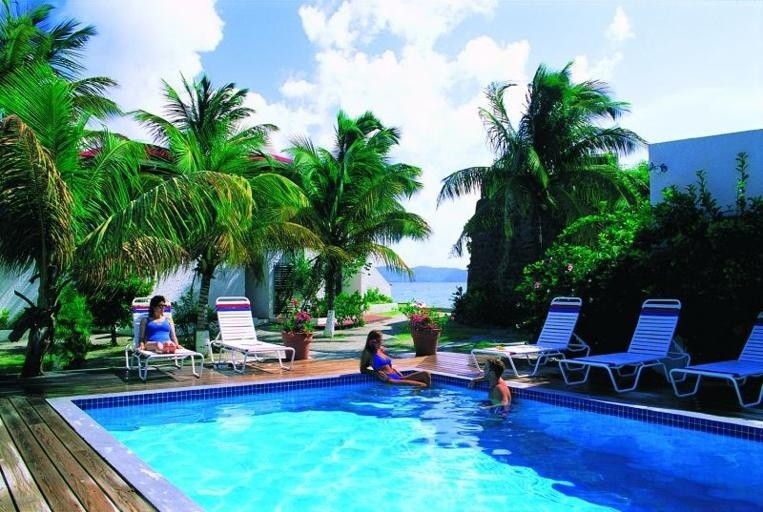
[359,329,432,389]
[467,356,512,419]
[136,295,182,354]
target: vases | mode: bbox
[282,332,313,359]
[411,329,442,356]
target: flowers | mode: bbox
[282,298,316,332]
[396,297,454,331]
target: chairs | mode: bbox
[123,296,204,379]
[559,298,691,393]
[471,296,591,378]
[207,295,295,374]
[669,311,763,408]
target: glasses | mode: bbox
[156,304,168,309]
